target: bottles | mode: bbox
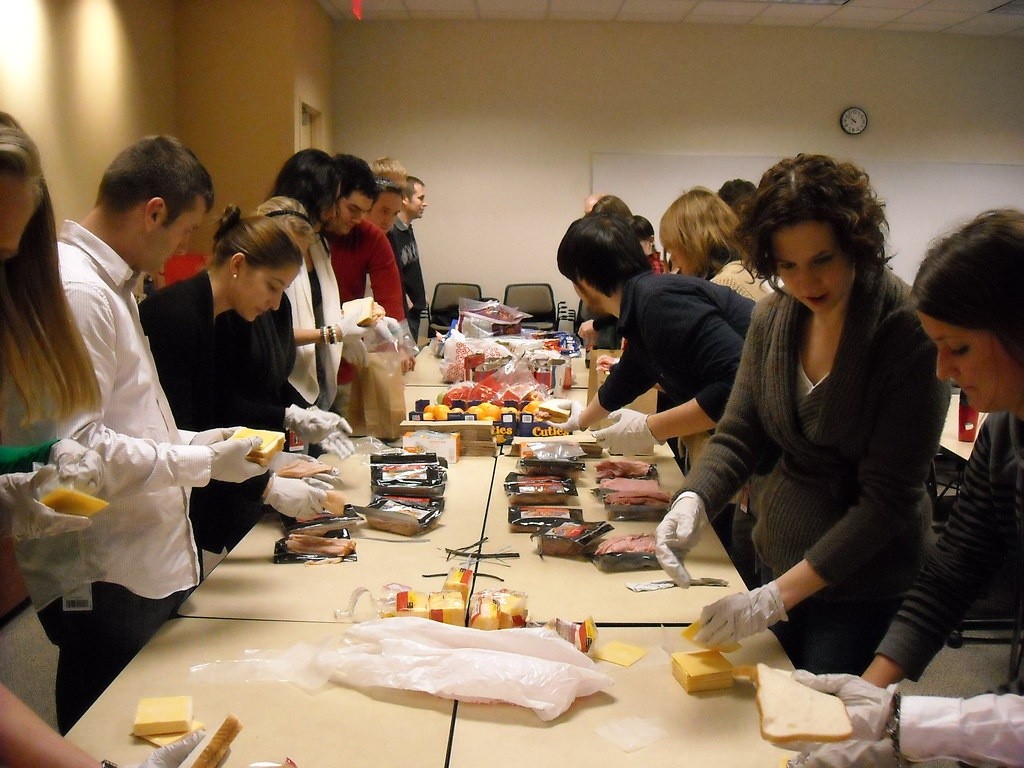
[558,349,571,390]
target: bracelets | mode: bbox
[885,690,901,753]
[321,325,337,343]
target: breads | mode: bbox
[226,427,286,465]
[753,661,853,743]
[538,399,570,422]
[342,296,374,326]
[176,713,243,768]
[277,460,334,478]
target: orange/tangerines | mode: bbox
[421,401,539,421]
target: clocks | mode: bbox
[840,107,867,134]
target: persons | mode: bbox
[0,111,428,762]
[534,151,1023,761]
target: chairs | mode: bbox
[924,456,1024,679]
[420,282,499,336]
[504,283,574,331]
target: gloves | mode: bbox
[191,426,246,445]
[344,338,368,368]
[304,406,354,460]
[210,435,271,484]
[591,409,667,459]
[38,439,107,496]
[0,464,94,539]
[285,403,340,444]
[771,669,900,743]
[786,738,913,768]
[654,499,706,589]
[273,452,340,489]
[693,582,789,651]
[543,399,584,432]
[374,316,400,343]
[264,472,334,519]
[336,315,374,343]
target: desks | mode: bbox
[942,391,988,463]
[62,339,816,768]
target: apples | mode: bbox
[529,392,543,401]
